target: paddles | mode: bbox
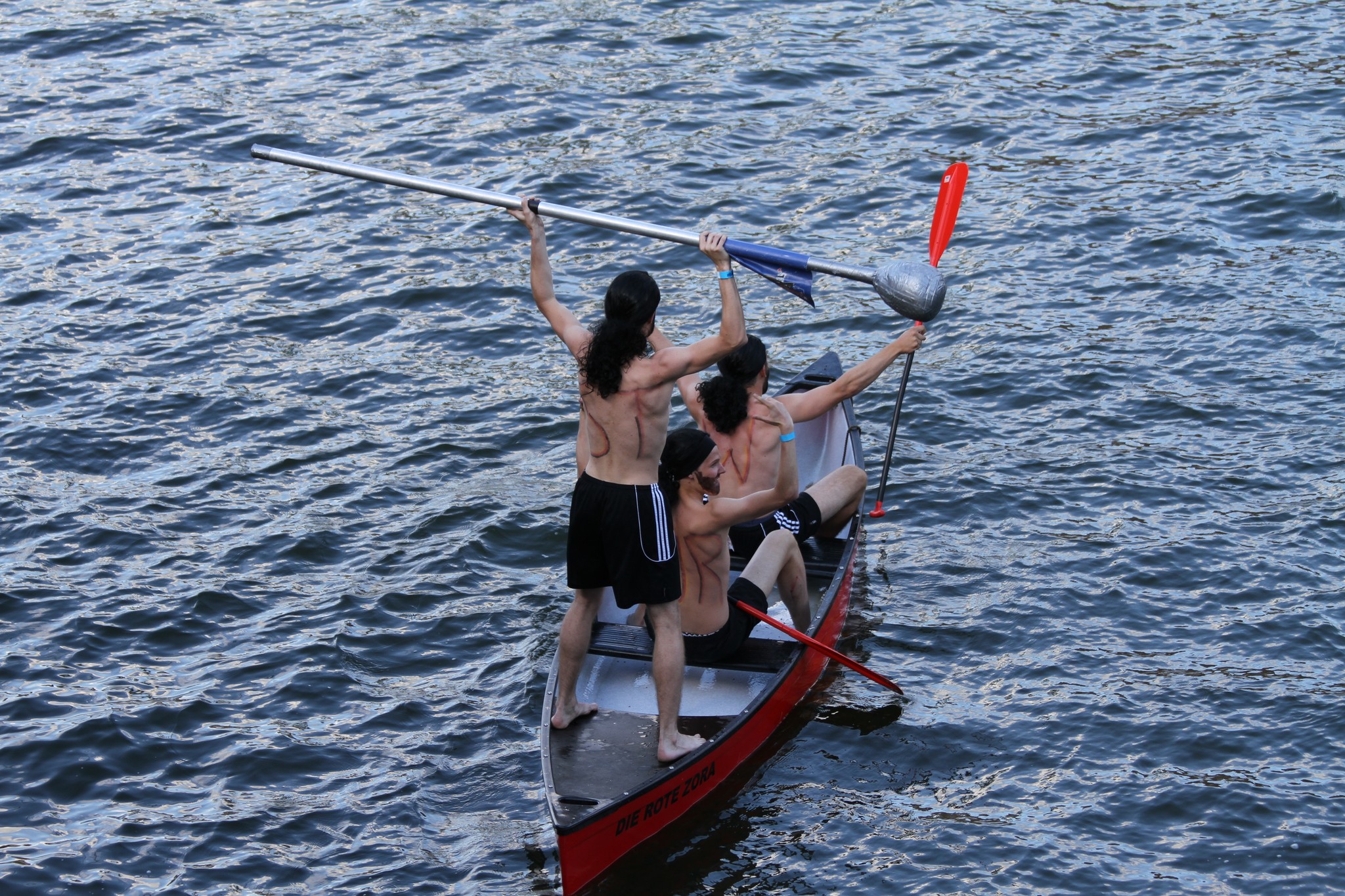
[869,162,970,518]
[728,595,906,698]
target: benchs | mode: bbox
[730,536,847,581]
[586,618,802,673]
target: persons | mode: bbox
[507,193,747,762]
[629,393,811,667]
[640,324,926,558]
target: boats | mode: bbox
[538,351,867,896]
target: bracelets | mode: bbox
[716,269,734,279]
[779,431,796,442]
